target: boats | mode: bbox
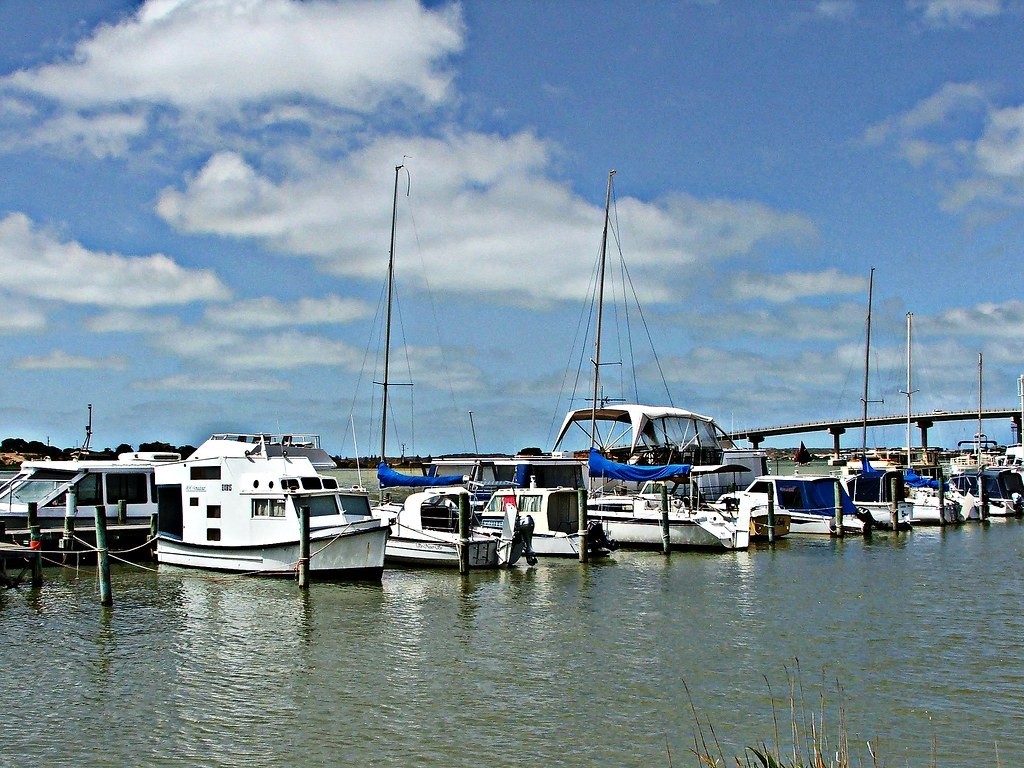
[847,452,894,469]
[0,404,181,543]
[156,433,392,580]
[745,475,864,534]
[422,457,620,557]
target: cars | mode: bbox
[932,410,948,415]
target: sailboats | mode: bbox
[337,164,536,568]
[550,168,753,548]
[947,353,1024,518]
[840,267,920,526]
[903,312,972,523]
